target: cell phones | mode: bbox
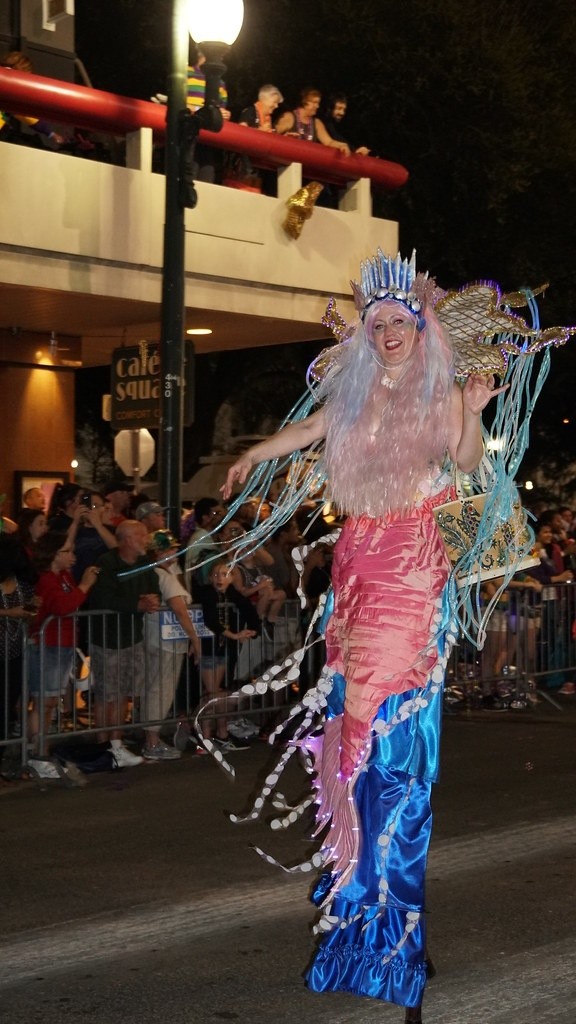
[80,494,92,509]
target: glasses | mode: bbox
[207,511,224,519]
[55,548,76,555]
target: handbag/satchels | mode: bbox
[432,455,542,590]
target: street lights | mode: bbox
[146,0,249,742]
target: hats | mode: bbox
[134,501,173,522]
[102,480,135,497]
[148,529,181,550]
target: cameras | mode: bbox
[91,567,102,575]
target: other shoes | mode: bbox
[557,682,576,694]
[27,714,276,788]
[233,634,266,680]
[442,682,545,715]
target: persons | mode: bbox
[219,286,512,1024]
[0,51,66,144]
[188,43,231,184]
[444,507,576,713]
[0,481,348,779]
[222,84,371,213]
